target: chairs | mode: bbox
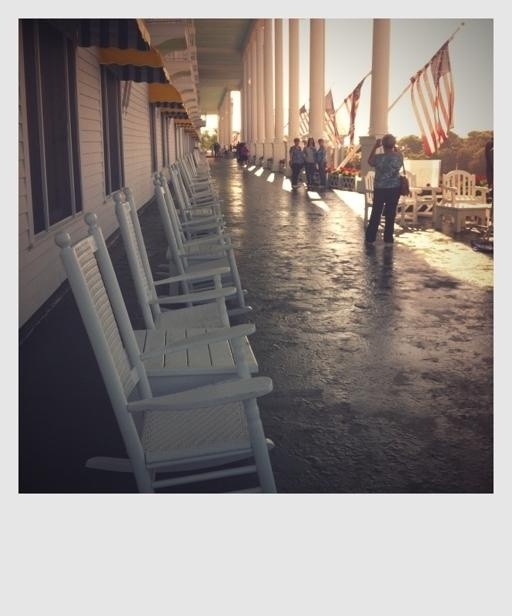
[363,170,490,224]
[153,149,251,317]
[54,211,276,494]
[115,187,235,327]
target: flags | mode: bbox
[343,82,361,146]
[325,88,343,149]
[409,39,455,158]
[299,106,310,136]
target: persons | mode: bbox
[366,134,404,246]
[239,143,249,166]
[289,138,303,190]
[303,138,317,191]
[214,141,220,158]
[317,139,327,192]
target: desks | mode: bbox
[128,325,260,390]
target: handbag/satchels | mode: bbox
[400,176,409,195]
